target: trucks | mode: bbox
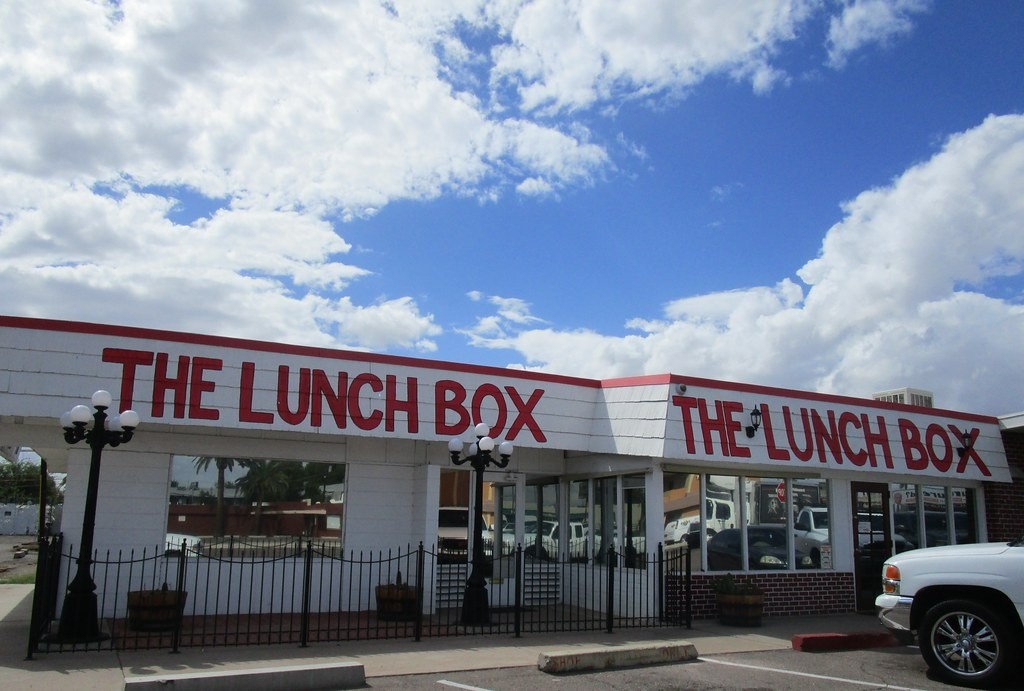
[525,520,646,560]
[664,515,700,544]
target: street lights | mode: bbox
[57,390,140,643]
[449,423,514,626]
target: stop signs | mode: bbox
[777,483,786,503]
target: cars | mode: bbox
[165,533,204,557]
[707,528,815,571]
[706,497,735,542]
[439,507,494,556]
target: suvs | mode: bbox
[794,505,914,569]
[873,533,1024,687]
